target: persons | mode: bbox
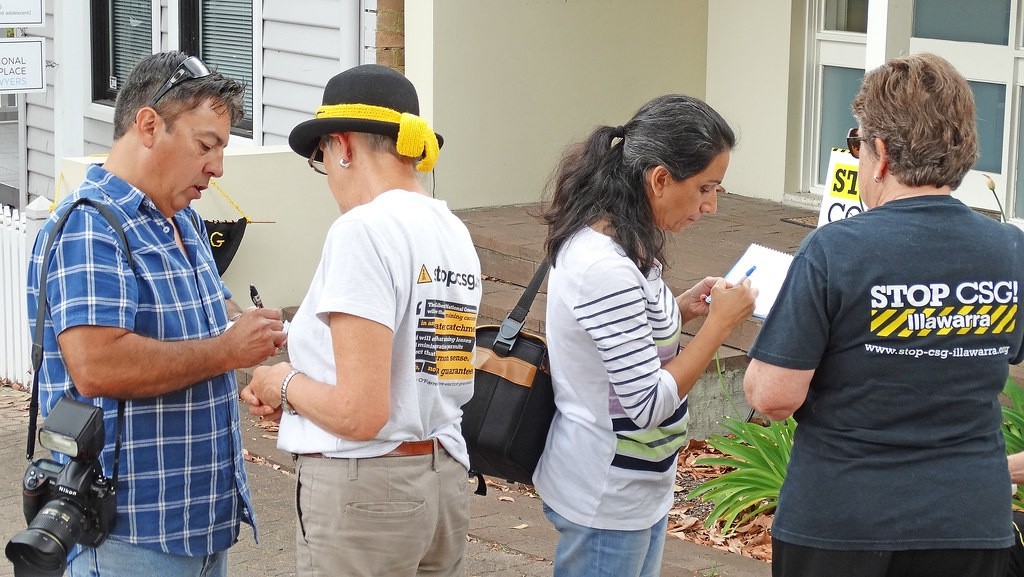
[26,51,289,576]
[532,93,759,576]
[240,64,480,576]
[742,52,1024,576]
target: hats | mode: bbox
[288,64,444,172]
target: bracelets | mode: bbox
[281,369,306,413]
[229,312,243,321]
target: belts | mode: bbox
[297,437,443,459]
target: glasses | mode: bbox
[135,55,212,124]
[307,137,327,175]
[847,128,875,160]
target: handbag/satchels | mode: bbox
[460,254,568,485]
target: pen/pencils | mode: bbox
[246,281,265,310]
[734,264,758,289]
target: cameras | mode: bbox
[4,397,119,577]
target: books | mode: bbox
[706,243,794,322]
